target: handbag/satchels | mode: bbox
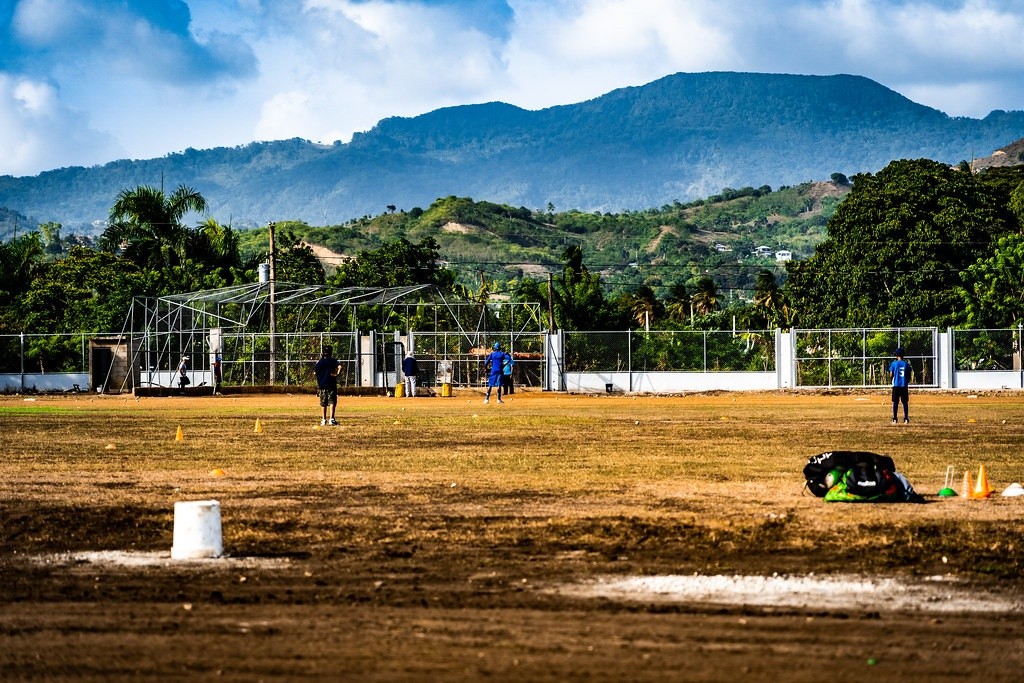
[803,450,922,503]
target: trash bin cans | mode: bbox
[605,383,613,393]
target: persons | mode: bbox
[213,358,221,396]
[402,352,420,397]
[314,345,342,426]
[484,343,504,404]
[500,351,514,396]
[177,356,189,396]
[888,348,910,423]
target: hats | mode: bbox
[324,345,333,352]
[183,356,190,360]
[896,348,904,355]
[494,342,500,349]
[217,357,223,360]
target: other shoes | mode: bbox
[321,419,327,425]
[904,418,909,423]
[329,418,339,425]
[498,399,504,403]
[484,399,489,404]
[893,418,898,423]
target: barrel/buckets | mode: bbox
[171,501,223,560]
[394,383,405,397]
[441,383,452,397]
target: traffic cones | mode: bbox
[174,424,185,444]
[973,461,992,499]
[254,416,265,433]
